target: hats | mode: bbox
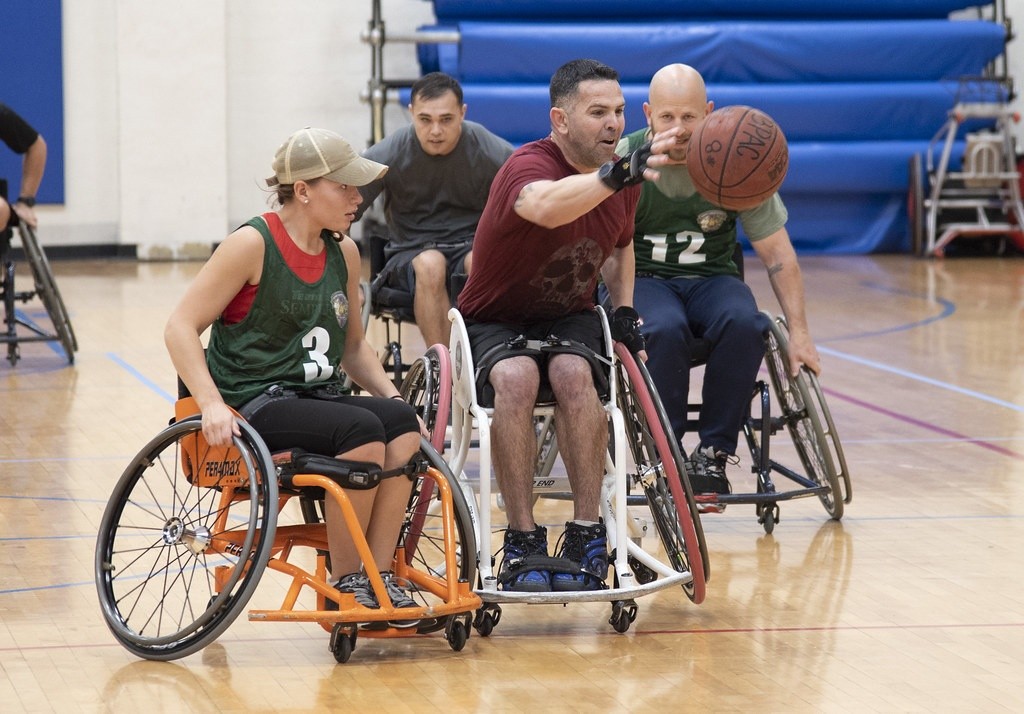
[266,127,387,188]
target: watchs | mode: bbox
[598,161,619,189]
[17,195,36,207]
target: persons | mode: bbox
[346,68,520,347]
[455,58,685,593]
[613,61,822,514]
[0,102,47,257]
[164,126,430,629]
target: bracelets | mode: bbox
[390,396,405,402]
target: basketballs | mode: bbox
[684,104,789,212]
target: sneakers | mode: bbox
[491,523,552,591]
[366,570,421,628]
[685,442,741,514]
[551,516,606,591]
[327,563,381,609]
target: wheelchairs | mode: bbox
[0,180,82,369]
[402,308,712,634]
[496,307,851,534]
[94,346,477,664]
[361,235,484,447]
